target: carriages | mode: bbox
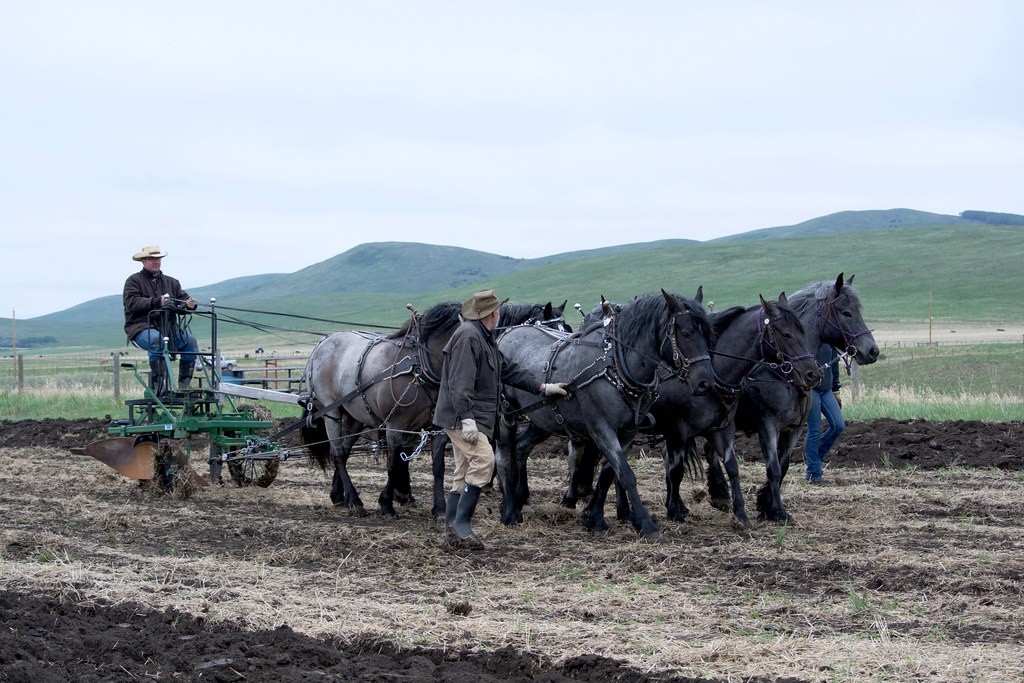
[82,272,880,542]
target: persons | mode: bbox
[805,343,845,482]
[432,289,572,543]
[123,243,197,391]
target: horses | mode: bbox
[299,270,881,537]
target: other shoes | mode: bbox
[807,476,830,486]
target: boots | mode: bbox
[178,354,195,397]
[444,480,484,551]
[149,358,167,399]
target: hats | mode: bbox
[132,246,165,261]
[459,288,510,320]
